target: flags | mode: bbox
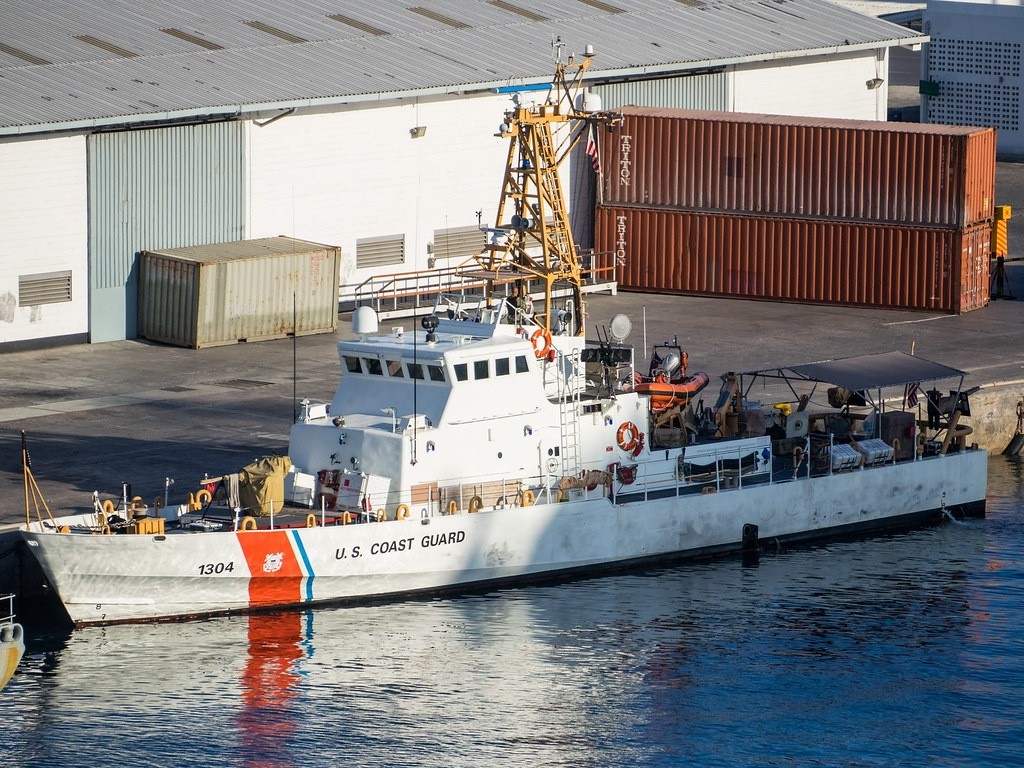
[908,381,919,408]
[586,125,604,177]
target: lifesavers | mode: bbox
[616,421,639,452]
[530,328,552,358]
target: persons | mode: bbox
[506,287,527,325]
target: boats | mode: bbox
[622,335,710,410]
[0,34,989,626]
[0,591,29,692]
